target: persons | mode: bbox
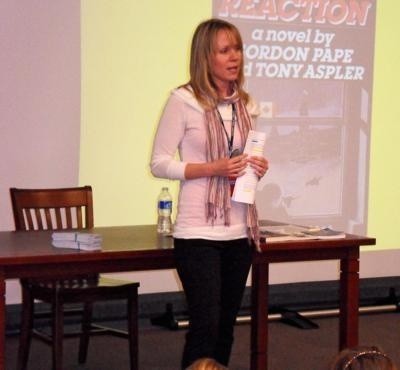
[149,17,268,370]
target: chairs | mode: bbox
[10,185,139,370]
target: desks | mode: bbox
[1,219,376,370]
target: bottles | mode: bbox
[156,186,173,236]
[298,89,310,116]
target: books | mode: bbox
[258,224,346,244]
